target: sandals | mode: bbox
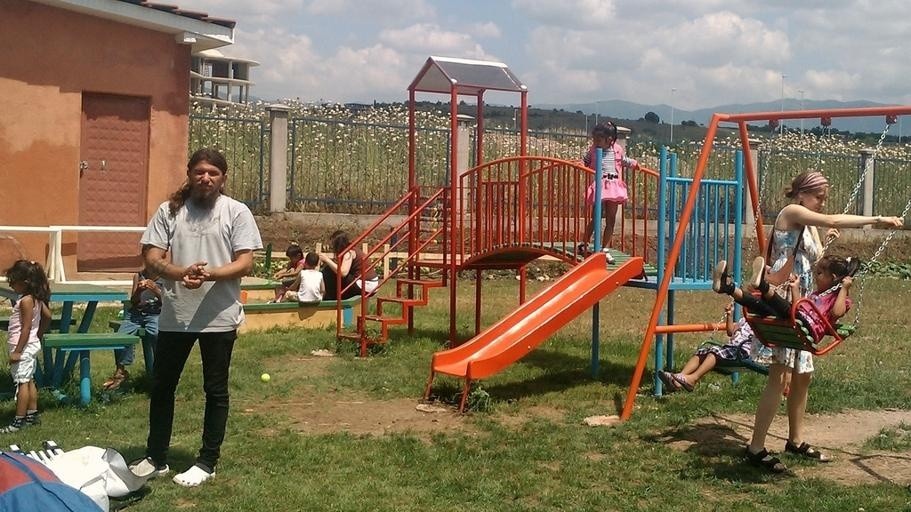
[0,412,39,433]
[785,440,830,462]
[578,243,614,263]
[746,445,788,473]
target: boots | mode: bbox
[713,260,735,295]
[751,256,770,294]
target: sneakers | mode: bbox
[103,371,128,391]
[659,370,694,393]
[172,465,215,488]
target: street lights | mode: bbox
[670,87,678,142]
[595,100,601,125]
[781,74,788,138]
[797,89,805,136]
[514,108,519,128]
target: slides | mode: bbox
[425,252,644,412]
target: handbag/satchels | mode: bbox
[770,273,792,302]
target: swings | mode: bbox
[694,126,833,387]
[744,122,911,355]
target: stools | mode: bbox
[0,278,154,405]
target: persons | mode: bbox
[267,230,379,306]
[103,245,166,392]
[576,120,642,263]
[0,260,52,434]
[131,147,264,487]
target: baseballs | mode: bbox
[261,373,272,383]
